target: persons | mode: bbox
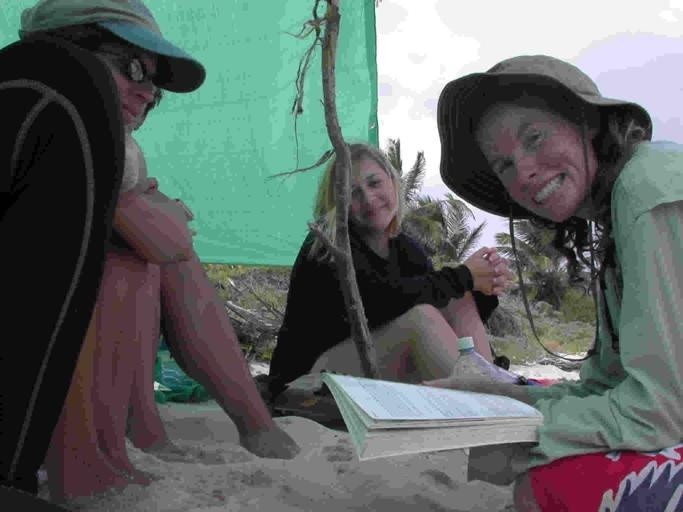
[268,144,510,422]
[1,1,302,512]
[421,55,683,512]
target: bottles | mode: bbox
[450,333,501,382]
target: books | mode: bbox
[285,371,545,464]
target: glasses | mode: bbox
[98,50,162,99]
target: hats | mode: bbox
[437,56,652,219]
[19,0,205,92]
